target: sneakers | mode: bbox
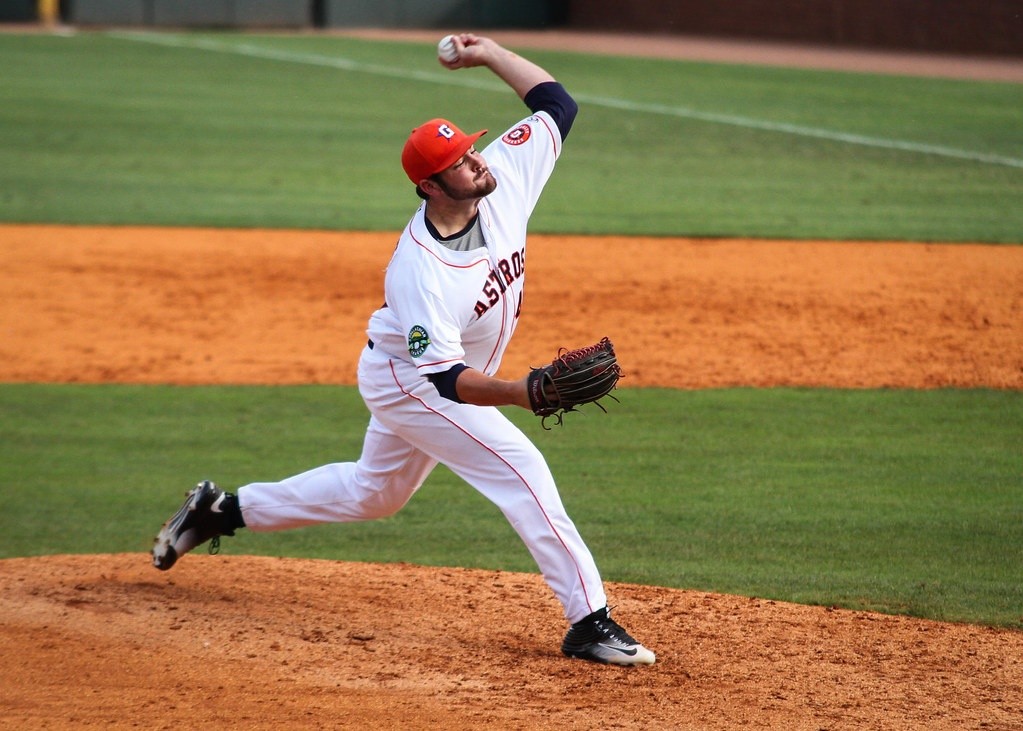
[152,479,235,571]
[562,606,657,666]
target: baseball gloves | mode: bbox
[527,338,621,415]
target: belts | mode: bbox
[367,338,374,349]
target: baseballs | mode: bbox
[438,35,465,63]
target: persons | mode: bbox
[150,32,657,666]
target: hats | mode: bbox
[401,118,488,186]
